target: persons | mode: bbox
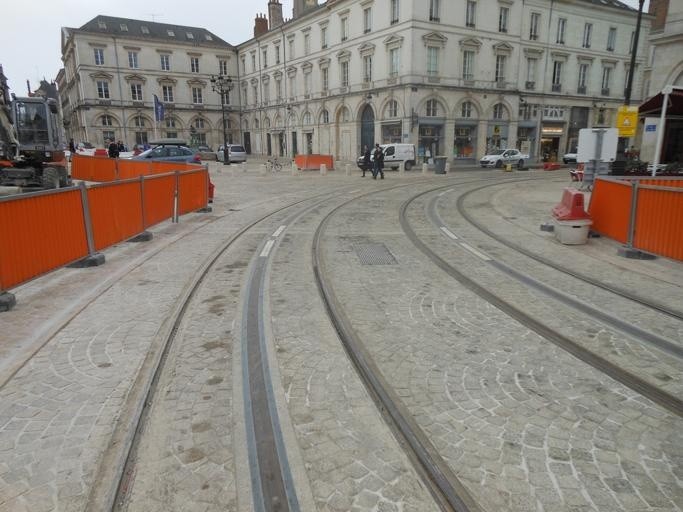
[104,137,149,157]
[67,138,76,162]
[371,148,385,179]
[372,143,379,179]
[360,144,373,177]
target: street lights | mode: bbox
[210,73,235,165]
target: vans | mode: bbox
[215,144,247,165]
[355,143,415,171]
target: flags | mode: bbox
[152,94,163,122]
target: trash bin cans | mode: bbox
[433,156,448,174]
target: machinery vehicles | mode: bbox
[0,66,73,199]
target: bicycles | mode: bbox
[265,156,282,173]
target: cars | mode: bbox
[563,152,577,164]
[123,145,201,167]
[478,148,524,168]
